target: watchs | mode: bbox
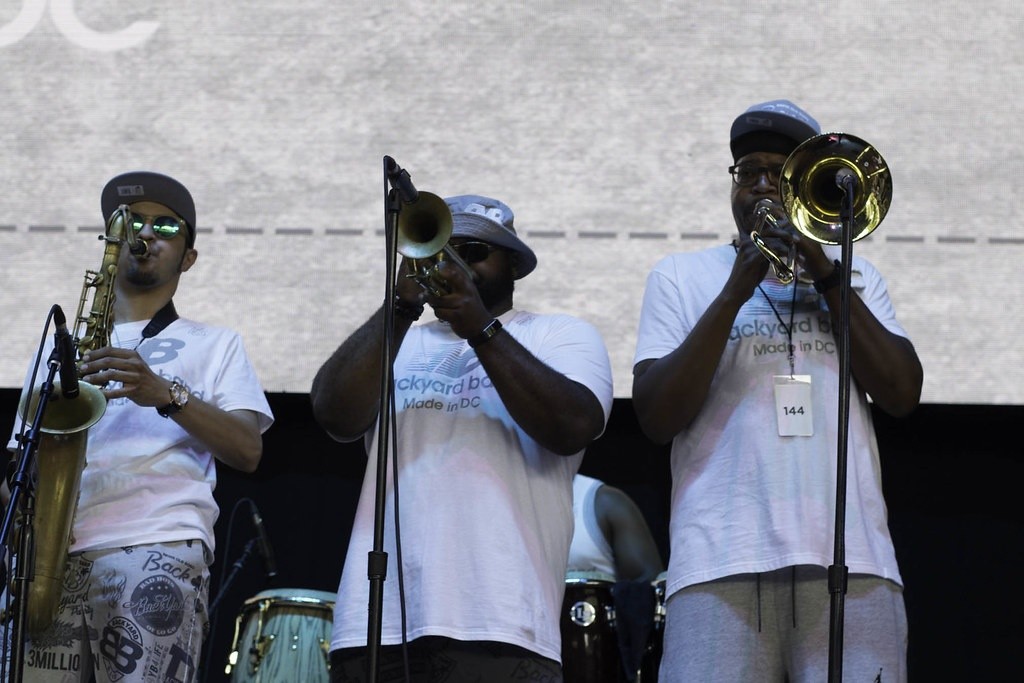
[156,381,188,419]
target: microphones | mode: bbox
[53,305,80,399]
[384,156,418,204]
[250,501,277,576]
[836,168,856,192]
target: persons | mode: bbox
[569,474,664,683]
[310,194,613,683]
[632,100,924,683]
[0,172,274,683]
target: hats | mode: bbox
[730,131,799,164]
[101,171,197,249]
[442,196,538,281]
[730,99,822,143]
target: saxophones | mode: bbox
[1,202,149,633]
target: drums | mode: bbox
[226,587,338,683]
[560,577,667,683]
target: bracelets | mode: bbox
[469,320,501,346]
[813,259,842,293]
[387,289,424,319]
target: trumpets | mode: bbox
[393,191,474,302]
[751,132,894,285]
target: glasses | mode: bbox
[432,242,497,263]
[129,211,190,241]
[728,162,780,187]
